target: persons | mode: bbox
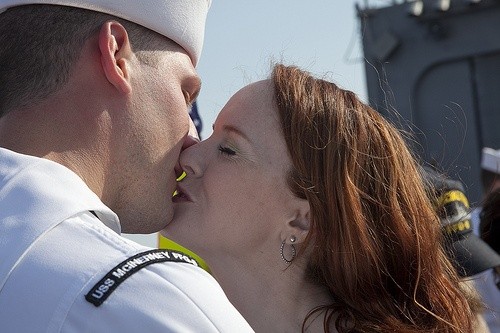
[462,186,500,333]
[470,147,500,237]
[0,0,257,333]
[160,64,490,333]
[422,167,500,278]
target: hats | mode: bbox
[0,0,212,68]
[481,147,500,173]
[423,174,500,277]
